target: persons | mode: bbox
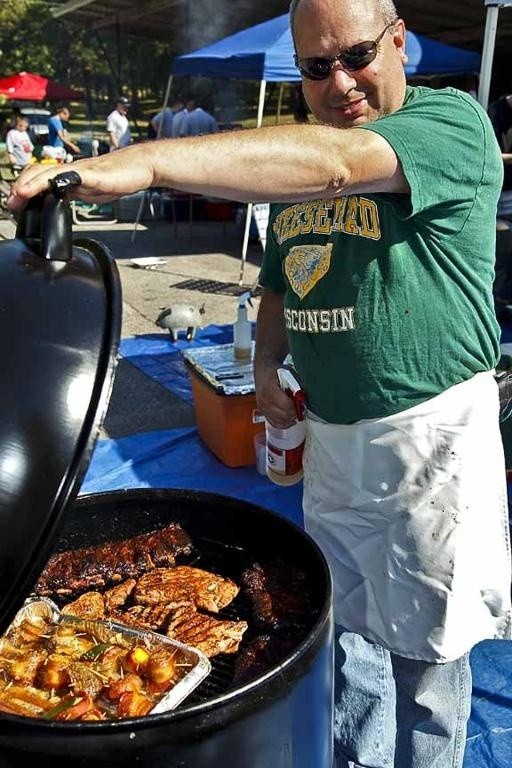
[8,1,512,767]
[3,90,217,221]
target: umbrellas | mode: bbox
[1,70,84,101]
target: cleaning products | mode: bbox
[233,291,254,359]
[265,369,306,487]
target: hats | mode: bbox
[117,96,131,108]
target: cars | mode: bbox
[14,106,54,141]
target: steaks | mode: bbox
[34,524,248,658]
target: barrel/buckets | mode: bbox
[254,432,266,475]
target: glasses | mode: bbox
[291,15,401,82]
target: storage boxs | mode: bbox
[180,339,297,470]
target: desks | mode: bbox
[157,187,230,246]
